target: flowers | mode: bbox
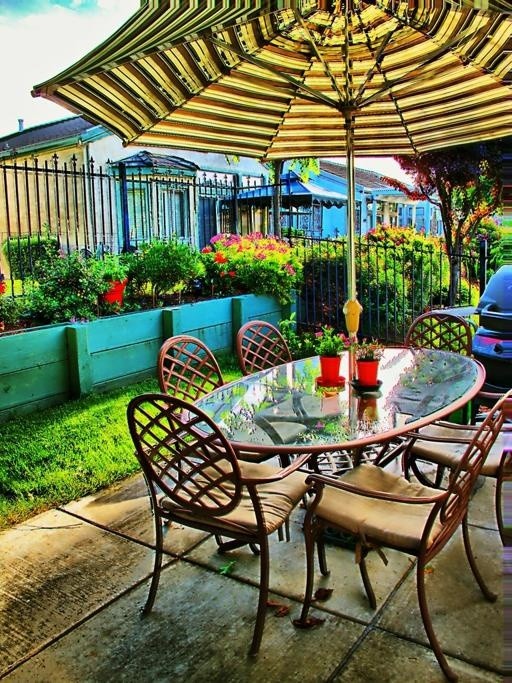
[301,322,357,357]
[355,337,388,361]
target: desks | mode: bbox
[179,347,487,512]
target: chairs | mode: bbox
[402,390,512,548]
[233,319,312,444]
[401,312,476,421]
[156,334,308,544]
[126,392,334,663]
[293,390,511,683]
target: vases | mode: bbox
[318,356,343,381]
[354,358,382,388]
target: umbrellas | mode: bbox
[28,0,511,383]
